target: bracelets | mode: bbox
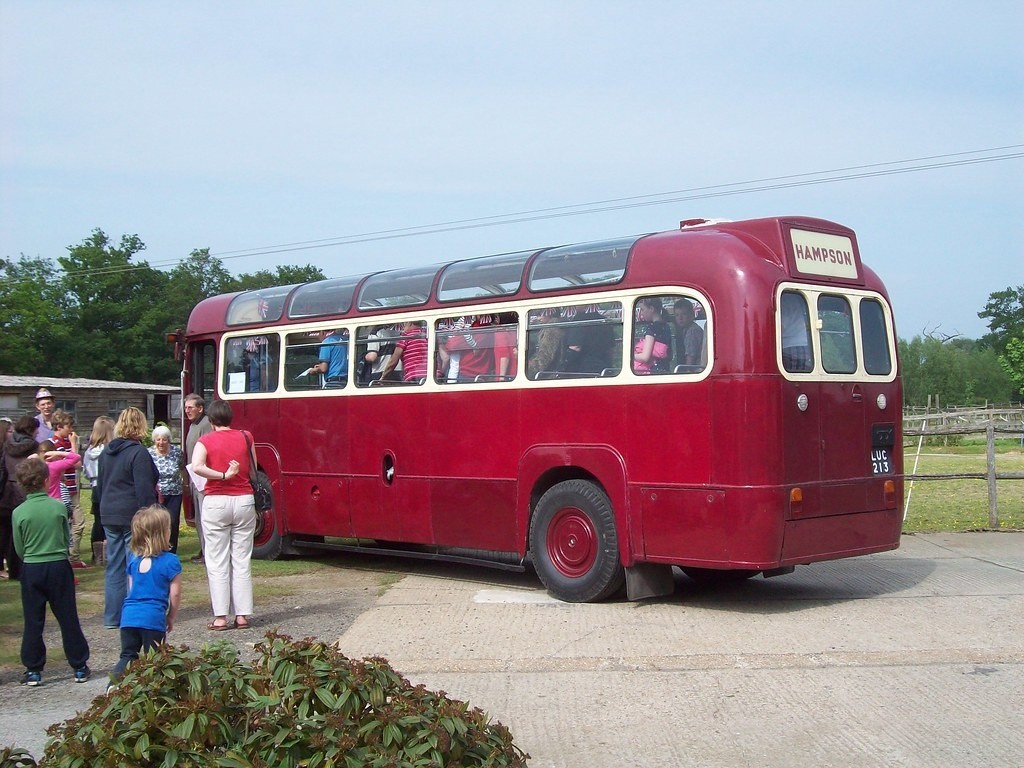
[183,485,188,487]
[223,472,225,479]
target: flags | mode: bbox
[231,336,269,352]
[258,300,268,316]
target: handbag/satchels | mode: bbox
[239,430,272,511]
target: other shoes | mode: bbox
[71,561,94,569]
[107,682,120,698]
[0,570,9,579]
[74,577,79,584]
[191,549,205,564]
[105,623,122,628]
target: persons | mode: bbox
[97,407,159,629]
[514,303,614,379]
[83,416,115,564]
[445,314,517,384]
[50,409,92,569]
[636,297,702,374]
[186,394,213,564]
[1,388,79,584]
[310,329,348,390]
[107,505,182,691]
[364,321,448,382]
[191,399,258,631]
[147,426,188,554]
[11,460,91,685]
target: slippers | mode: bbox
[234,615,249,629]
[206,617,228,630]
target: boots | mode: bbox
[103,539,108,566]
[91,541,103,566]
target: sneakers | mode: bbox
[73,665,92,681]
[21,668,42,685]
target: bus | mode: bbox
[167,215,906,604]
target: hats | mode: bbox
[0,417,14,425]
[36,388,55,399]
